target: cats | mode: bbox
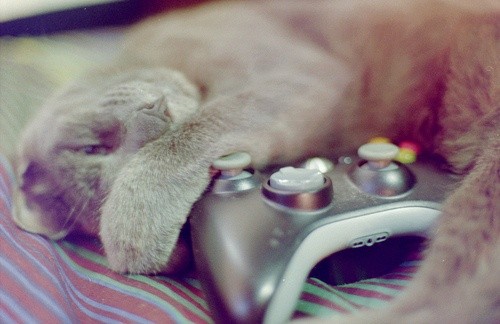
[9,0,500,324]
